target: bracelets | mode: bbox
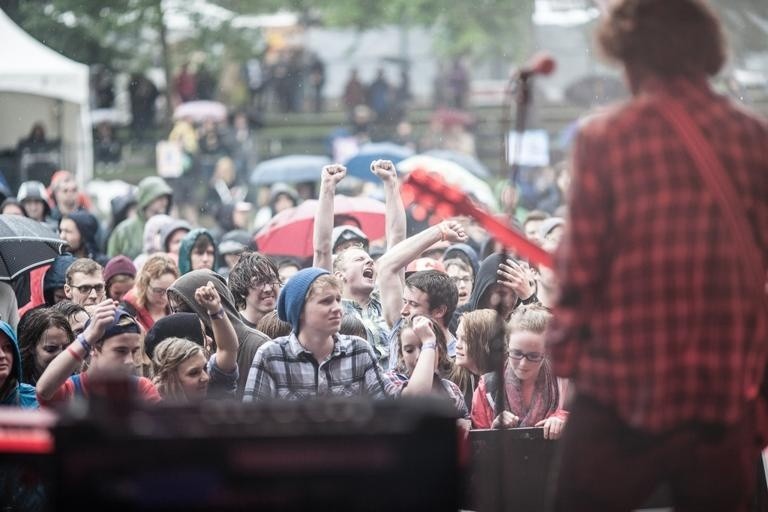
[420,343,436,350]
[68,346,83,363]
[554,409,570,419]
[208,307,224,319]
[78,334,91,349]
[522,293,539,305]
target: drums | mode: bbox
[467,426,549,512]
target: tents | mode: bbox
[0,9,94,181]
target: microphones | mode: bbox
[510,52,557,84]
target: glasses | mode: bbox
[452,276,473,284]
[247,279,283,290]
[507,351,544,364]
[71,284,105,293]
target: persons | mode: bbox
[431,51,479,157]
[542,0,768,511]
[90,47,325,204]
[345,57,418,151]
[0,162,574,439]
[588,77,610,106]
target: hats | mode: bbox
[81,310,140,342]
[335,231,364,246]
[276,267,330,334]
